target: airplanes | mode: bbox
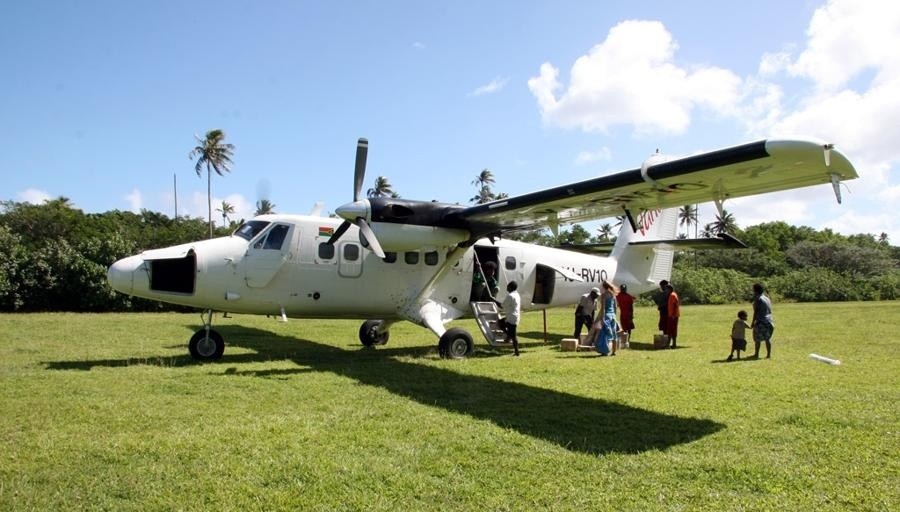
[107,137,860,361]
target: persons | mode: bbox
[492,280,521,356]
[664,284,681,349]
[573,287,602,345]
[746,282,776,358]
[652,279,668,330]
[594,280,624,356]
[472,261,501,302]
[726,310,752,360]
[616,283,635,342]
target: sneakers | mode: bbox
[728,355,733,361]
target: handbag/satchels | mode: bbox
[593,321,602,329]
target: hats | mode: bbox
[591,288,602,295]
